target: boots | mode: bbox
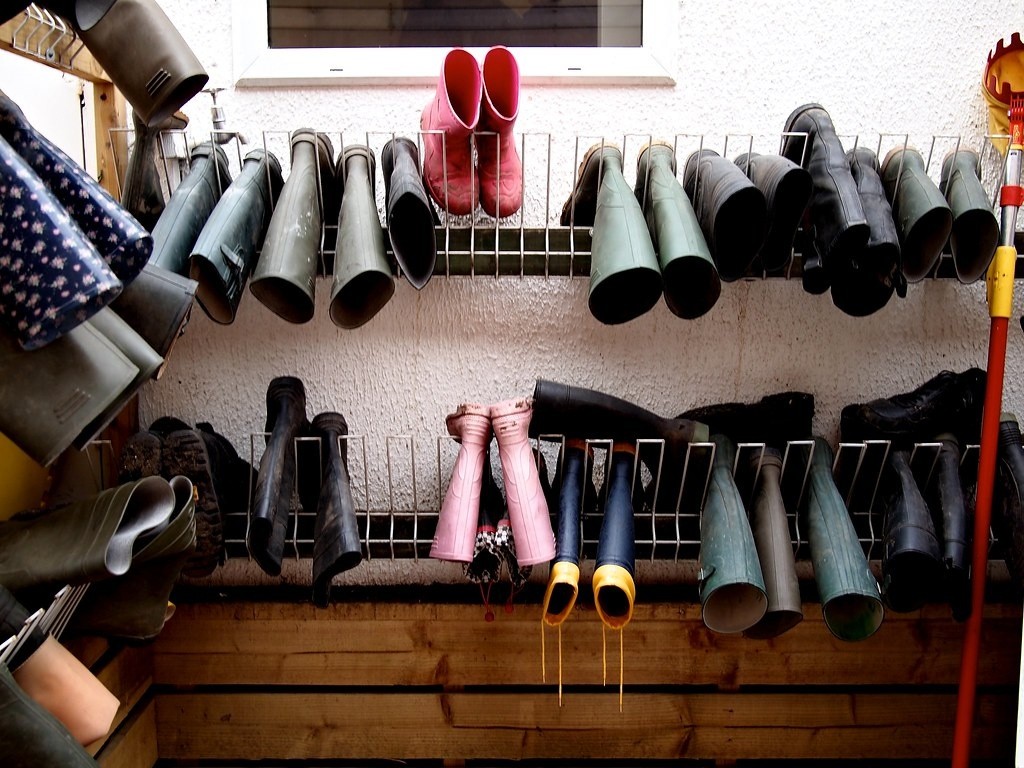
[0,90,200,468]
[123,47,999,330]
[0,0,209,127]
[0,377,1023,768]
